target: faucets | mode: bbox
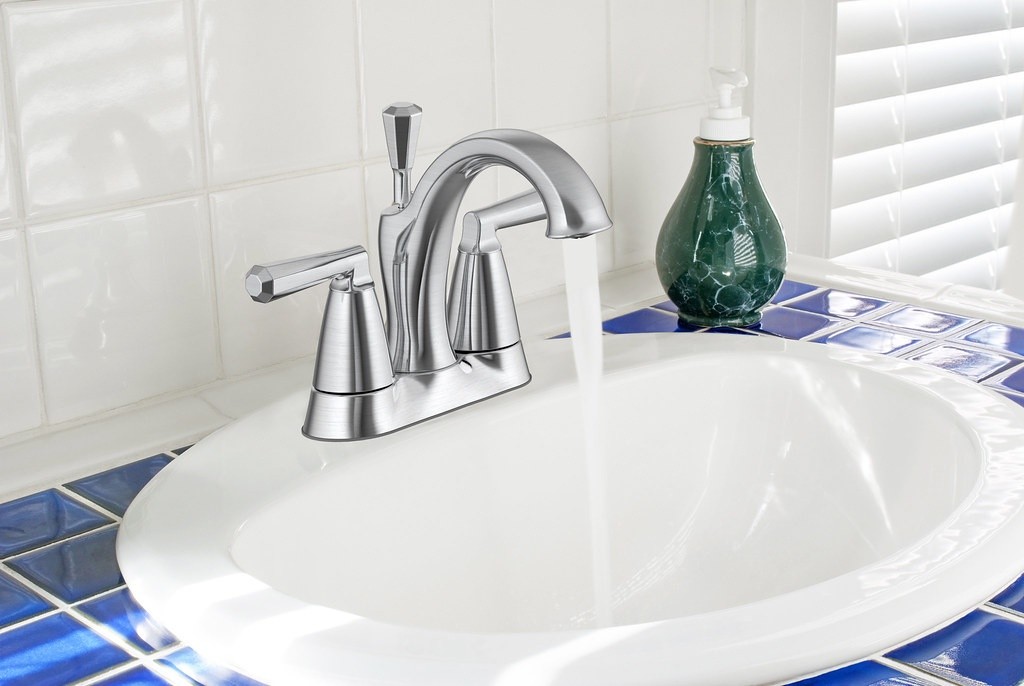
[391,129,613,375]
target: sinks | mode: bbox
[110,318,1024,686]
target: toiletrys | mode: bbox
[653,60,790,328]
